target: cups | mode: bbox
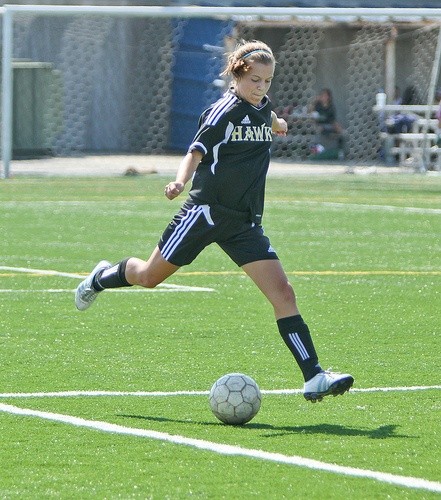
[376,93,387,105]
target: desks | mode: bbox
[269,112,350,159]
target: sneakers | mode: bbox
[303,367,354,403]
[76,261,112,311]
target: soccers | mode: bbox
[209,373,261,425]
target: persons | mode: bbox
[375,84,441,167]
[75,38,355,405]
[313,88,350,161]
